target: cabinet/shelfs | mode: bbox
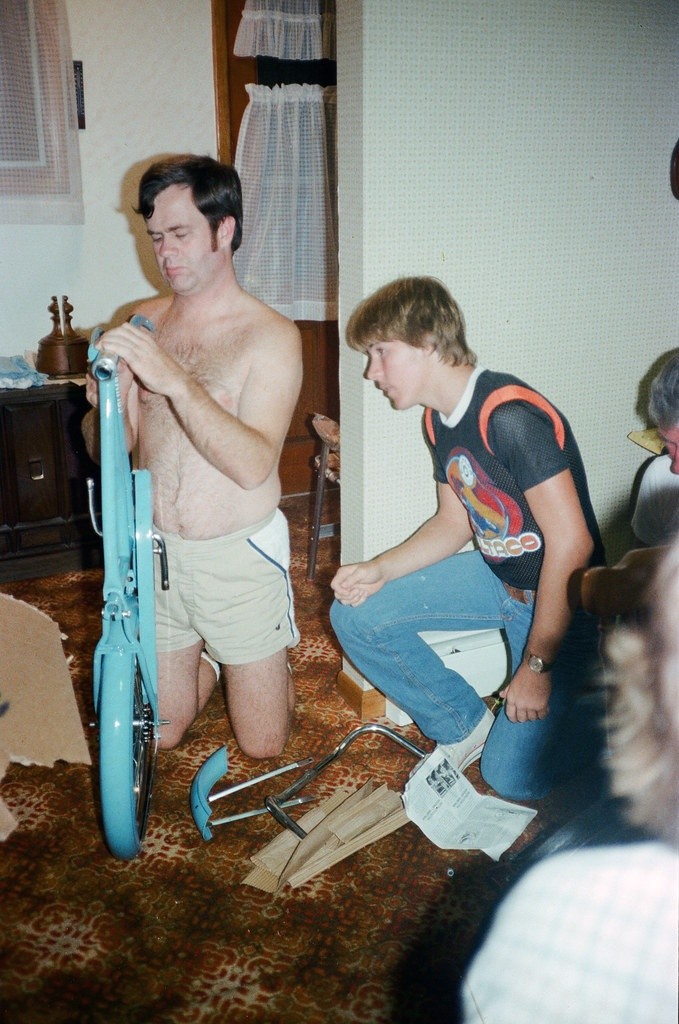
[0,383,104,584]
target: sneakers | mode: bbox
[410,708,496,782]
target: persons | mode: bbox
[461,354,679,1022]
[328,276,603,795]
[90,152,302,755]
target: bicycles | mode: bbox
[87,317,170,861]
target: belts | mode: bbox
[502,580,581,606]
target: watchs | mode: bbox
[524,647,554,675]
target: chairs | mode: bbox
[307,412,341,583]
